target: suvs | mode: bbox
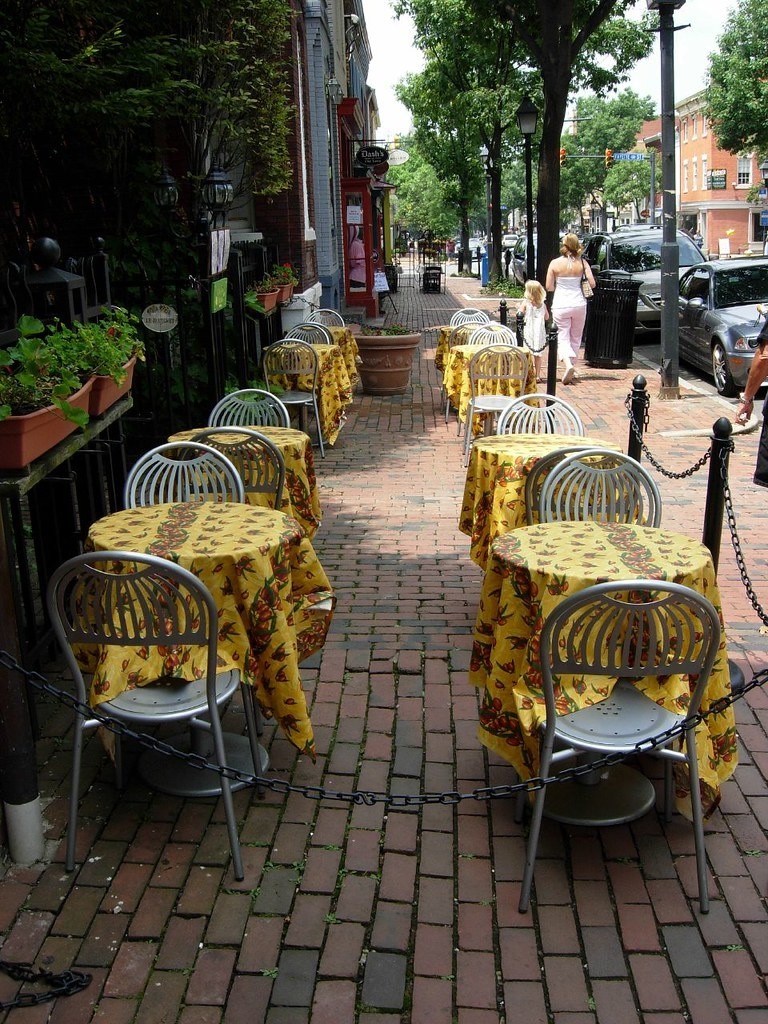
[581,223,709,335]
[512,235,564,286]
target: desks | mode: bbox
[162,428,323,544]
[262,343,354,449]
[467,521,739,829]
[442,344,539,434]
[433,326,477,373]
[301,327,363,385]
[458,434,646,574]
[68,501,335,798]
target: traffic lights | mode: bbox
[606,150,613,168]
[560,148,566,167]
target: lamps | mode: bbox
[732,182,737,186]
[326,76,345,105]
[345,13,359,24]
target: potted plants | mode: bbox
[1,302,144,471]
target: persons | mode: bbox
[517,279,549,381]
[734,314,768,489]
[348,226,367,287]
[546,233,595,384]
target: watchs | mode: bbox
[740,393,754,404]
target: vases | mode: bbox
[244,283,294,312]
[353,333,420,395]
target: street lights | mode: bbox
[478,144,493,241]
[590,200,597,234]
[514,92,535,280]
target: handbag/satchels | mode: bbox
[581,257,594,298]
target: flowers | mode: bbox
[357,321,411,336]
[245,262,299,294]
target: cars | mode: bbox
[454,238,486,259]
[679,256,768,397]
[501,234,519,252]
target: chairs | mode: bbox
[47,307,722,917]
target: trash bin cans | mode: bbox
[585,270,644,369]
[423,266,442,293]
[482,254,488,288]
[386,265,399,293]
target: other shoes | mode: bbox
[562,366,579,385]
[536,378,542,383]
